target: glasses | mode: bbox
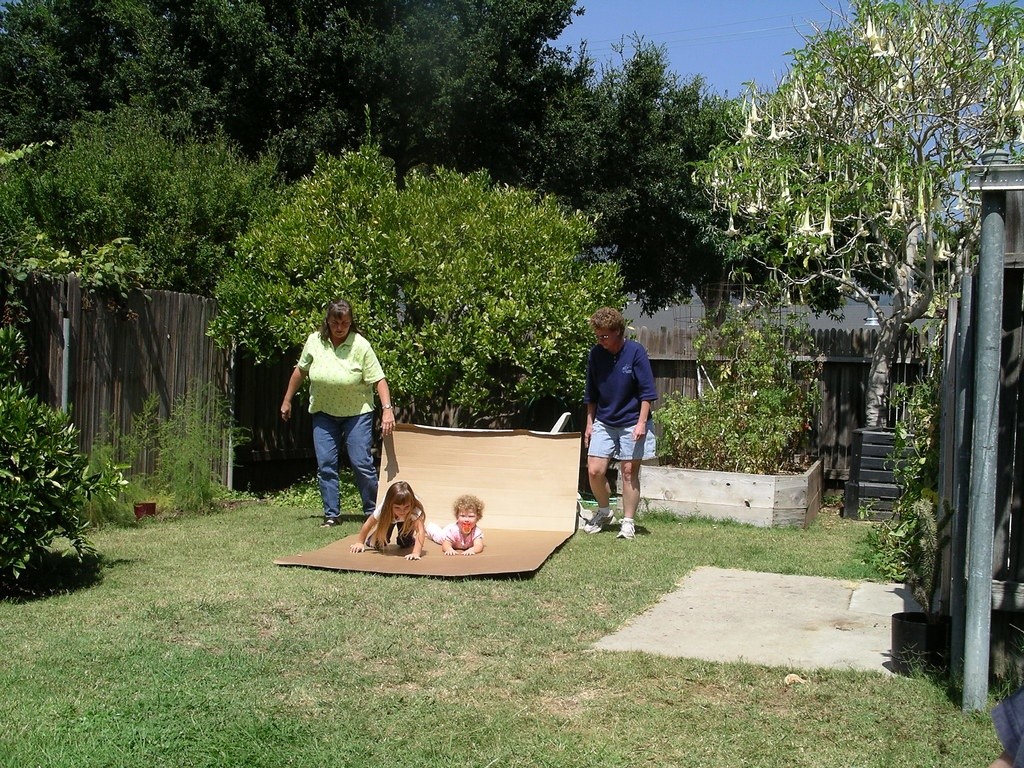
[595,331,615,340]
[327,320,351,327]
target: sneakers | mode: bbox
[616,517,636,540]
[584,509,614,535]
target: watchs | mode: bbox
[382,404,393,409]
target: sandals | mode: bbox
[319,516,343,528]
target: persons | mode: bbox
[350,480,426,560]
[583,307,658,540]
[424,494,485,555]
[280,299,396,528]
